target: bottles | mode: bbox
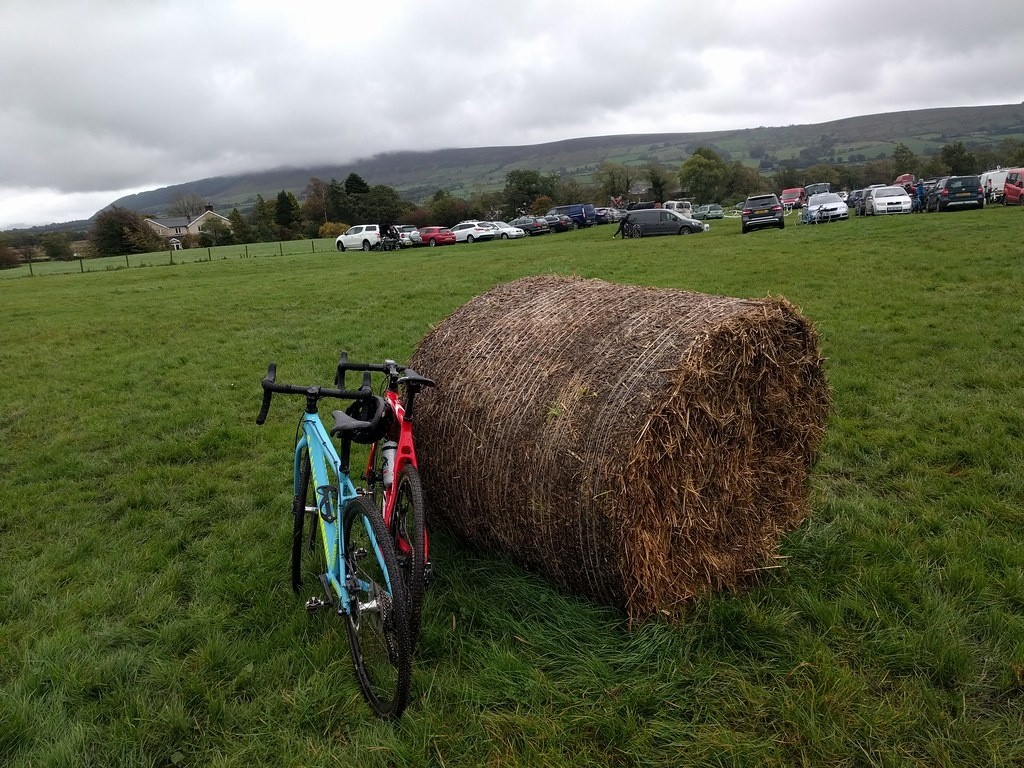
[381,440,398,488]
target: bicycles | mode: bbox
[255,361,411,723]
[332,353,437,667]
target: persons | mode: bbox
[916,179,926,214]
[987,178,992,204]
[613,214,634,239]
[904,183,912,194]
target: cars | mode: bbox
[335,162,1024,251]
